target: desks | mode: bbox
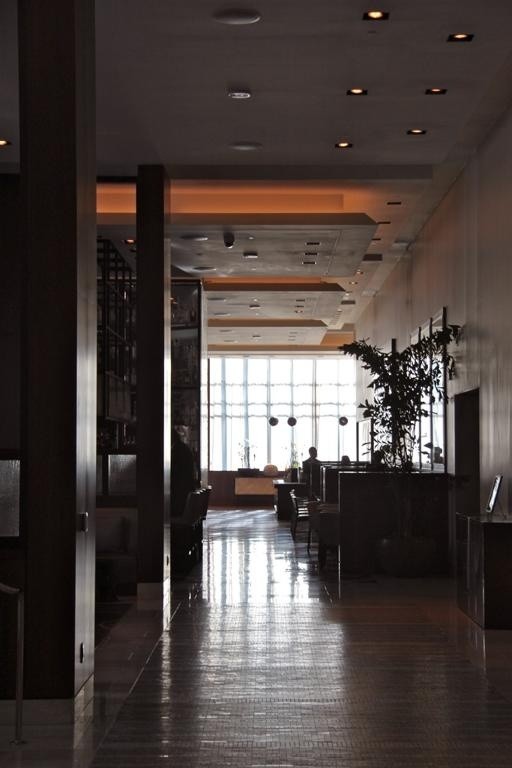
[272,479,306,520]
[235,476,278,506]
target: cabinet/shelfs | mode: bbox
[455,511,512,630]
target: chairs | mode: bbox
[289,487,337,567]
[168,484,213,579]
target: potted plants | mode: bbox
[238,438,259,477]
[282,441,303,482]
[337,320,462,576]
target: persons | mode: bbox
[302,446,322,464]
[170,428,195,513]
